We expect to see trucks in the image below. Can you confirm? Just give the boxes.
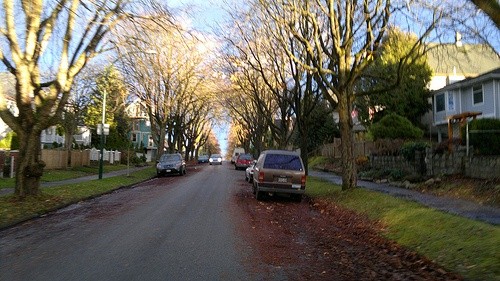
[231,148,245,164]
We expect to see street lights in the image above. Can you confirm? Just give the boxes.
[98,50,158,179]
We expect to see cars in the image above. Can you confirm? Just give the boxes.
[235,153,254,170]
[253,149,305,200]
[156,154,186,178]
[245,160,259,183]
[198,155,209,162]
[209,154,222,165]
